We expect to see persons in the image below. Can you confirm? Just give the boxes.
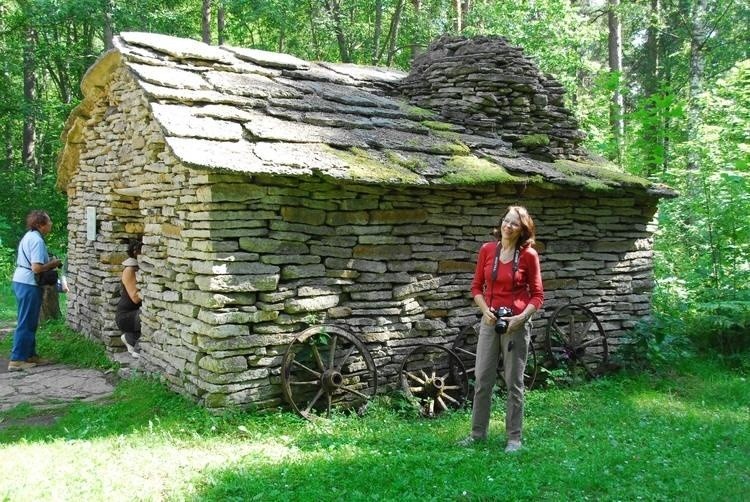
[7,209,61,371]
[114,237,144,359]
[454,204,545,454]
[61,252,69,293]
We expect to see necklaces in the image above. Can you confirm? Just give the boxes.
[499,246,513,261]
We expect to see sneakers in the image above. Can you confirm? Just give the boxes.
[7,356,49,372]
[121,333,141,359]
[503,440,523,455]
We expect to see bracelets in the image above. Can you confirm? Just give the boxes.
[522,310,530,320]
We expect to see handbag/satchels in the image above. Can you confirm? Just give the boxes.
[35,270,59,286]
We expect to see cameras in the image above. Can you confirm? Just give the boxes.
[494,306,514,334]
[56,256,64,269]
